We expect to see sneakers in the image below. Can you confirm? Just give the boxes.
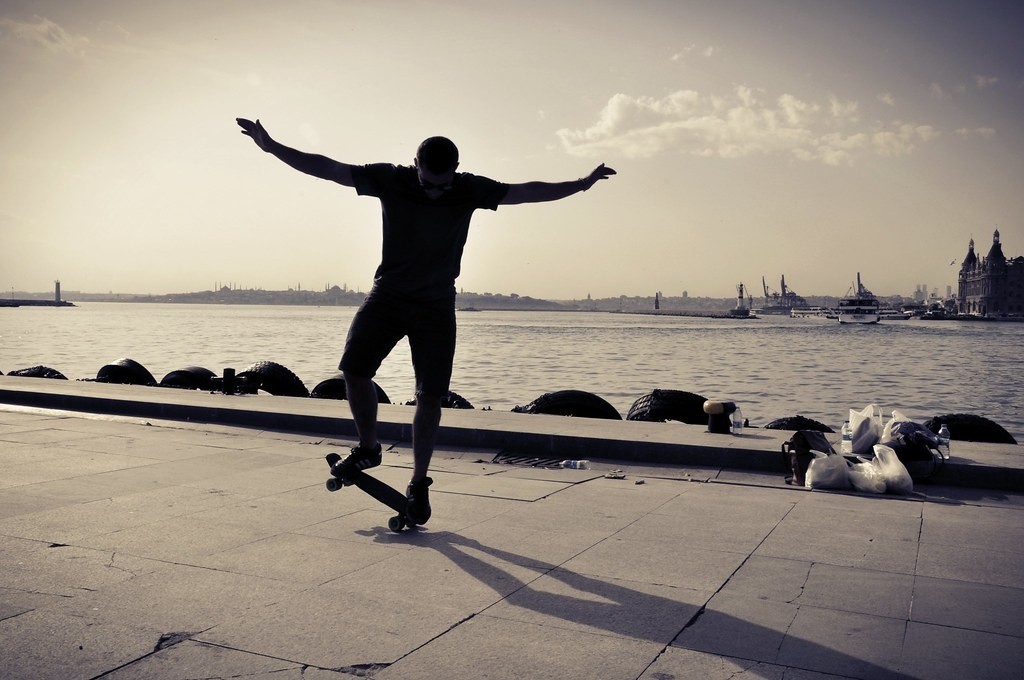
[406,477,433,523]
[330,443,382,478]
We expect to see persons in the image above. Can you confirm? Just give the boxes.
[237,118,620,522]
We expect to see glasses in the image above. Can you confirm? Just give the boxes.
[419,174,455,190]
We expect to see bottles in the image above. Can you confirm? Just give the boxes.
[558,460,591,471]
[732,407,743,435]
[937,424,950,459]
[841,421,853,453]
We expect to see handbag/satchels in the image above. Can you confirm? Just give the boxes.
[891,421,944,479]
[805,403,913,494]
[782,429,838,485]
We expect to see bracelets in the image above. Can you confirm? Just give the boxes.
[576,177,588,192]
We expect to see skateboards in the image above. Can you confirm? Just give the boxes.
[326,452,418,532]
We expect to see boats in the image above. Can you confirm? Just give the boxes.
[836,270,881,326]
[789,305,837,322]
[879,307,912,321]
